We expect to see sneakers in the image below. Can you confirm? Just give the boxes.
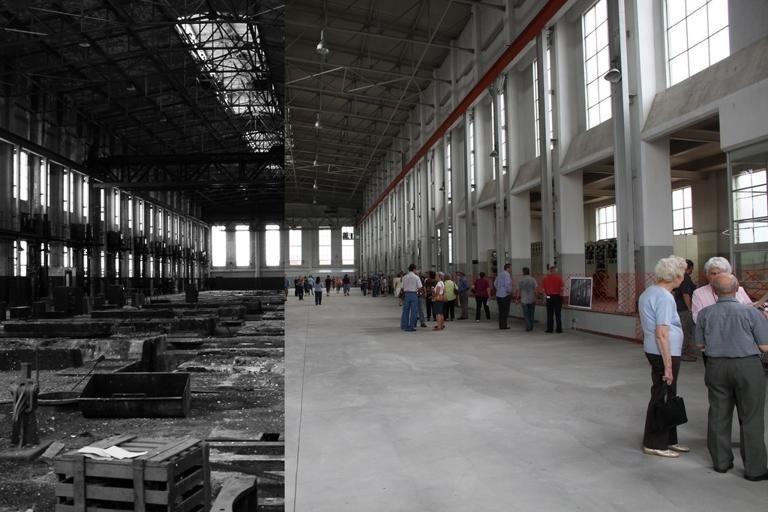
[412,318,444,331]
[500,327,511,329]
[446,317,466,321]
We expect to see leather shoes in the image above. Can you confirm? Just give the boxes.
[715,462,734,473]
[642,446,679,457]
[668,444,690,452]
[744,474,768,481]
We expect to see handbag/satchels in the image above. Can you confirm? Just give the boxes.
[432,294,448,302]
[652,396,688,431]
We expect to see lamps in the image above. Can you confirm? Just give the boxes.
[315,113,322,128]
[79,37,90,47]
[160,115,167,121]
[314,156,320,166]
[313,179,319,189]
[126,83,136,91]
[317,30,329,53]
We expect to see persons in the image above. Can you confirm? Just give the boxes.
[285,260,566,335]
[637,254,690,458]
[693,272,768,483]
[674,258,698,362]
[691,255,768,328]
